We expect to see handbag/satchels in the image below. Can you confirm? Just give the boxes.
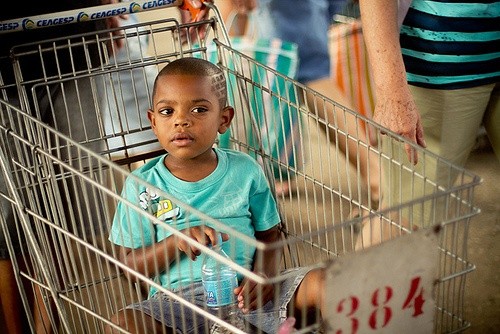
[197,7,306,180]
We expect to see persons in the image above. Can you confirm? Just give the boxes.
[230,0,386,233]
[0,0,210,334]
[354,0,500,251]
[105,56,328,334]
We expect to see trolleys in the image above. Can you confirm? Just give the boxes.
[0,0,486,334]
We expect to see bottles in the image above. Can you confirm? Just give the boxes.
[199,232,251,334]
